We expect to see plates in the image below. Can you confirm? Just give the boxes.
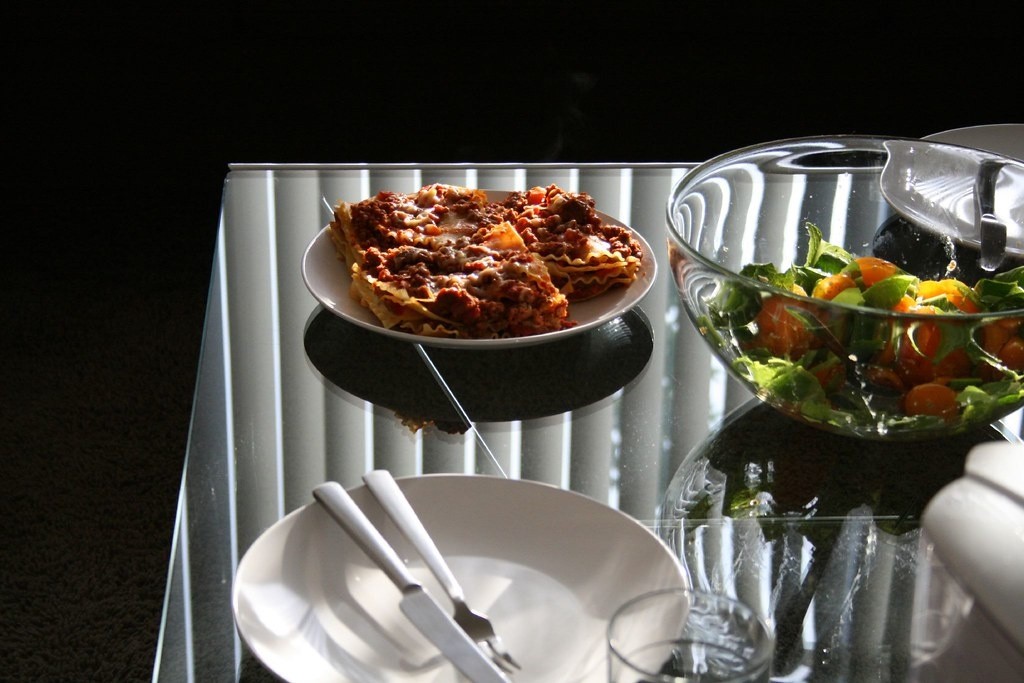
[230,474,687,682]
[303,191,657,345]
[880,122,1024,258]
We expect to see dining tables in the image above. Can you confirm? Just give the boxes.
[152,162,1023,683]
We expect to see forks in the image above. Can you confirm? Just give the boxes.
[362,470,520,675]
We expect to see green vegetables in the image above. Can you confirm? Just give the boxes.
[693,218,1024,443]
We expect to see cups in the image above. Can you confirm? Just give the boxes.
[607,588,772,682]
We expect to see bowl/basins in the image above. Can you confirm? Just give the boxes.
[666,136,1024,440]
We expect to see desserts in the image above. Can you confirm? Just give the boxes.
[325,184,647,340]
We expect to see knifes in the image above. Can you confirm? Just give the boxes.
[310,482,509,682]
[976,158,1007,272]
